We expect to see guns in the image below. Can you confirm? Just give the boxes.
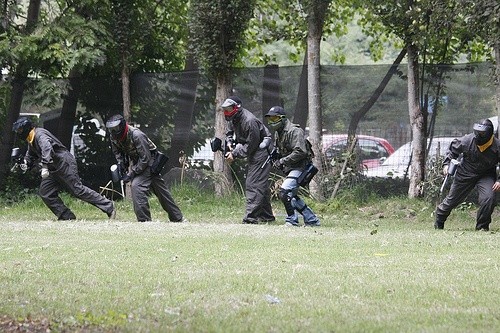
[110,157,125,198]
[439,158,459,193]
[258,135,277,169]
[211,130,236,159]
[9,147,32,175]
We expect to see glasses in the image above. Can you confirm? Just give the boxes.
[108,123,122,132]
[14,127,24,133]
[224,105,234,115]
[266,116,282,126]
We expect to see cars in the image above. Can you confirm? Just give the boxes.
[305,134,395,196]
[367,137,463,185]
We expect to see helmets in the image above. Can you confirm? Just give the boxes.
[11,117,34,141]
[222,97,242,121]
[266,106,286,131]
[106,114,126,139]
[473,119,493,146]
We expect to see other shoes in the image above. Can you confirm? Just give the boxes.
[304,221,321,227]
[109,201,116,219]
[434,220,444,230]
[285,222,293,226]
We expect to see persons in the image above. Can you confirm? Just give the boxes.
[11,117,116,221]
[211,96,275,224]
[259,106,320,227]
[106,114,188,223]
[433,118,500,230]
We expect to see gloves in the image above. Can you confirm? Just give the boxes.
[272,160,284,170]
[39,168,49,179]
[121,173,131,183]
